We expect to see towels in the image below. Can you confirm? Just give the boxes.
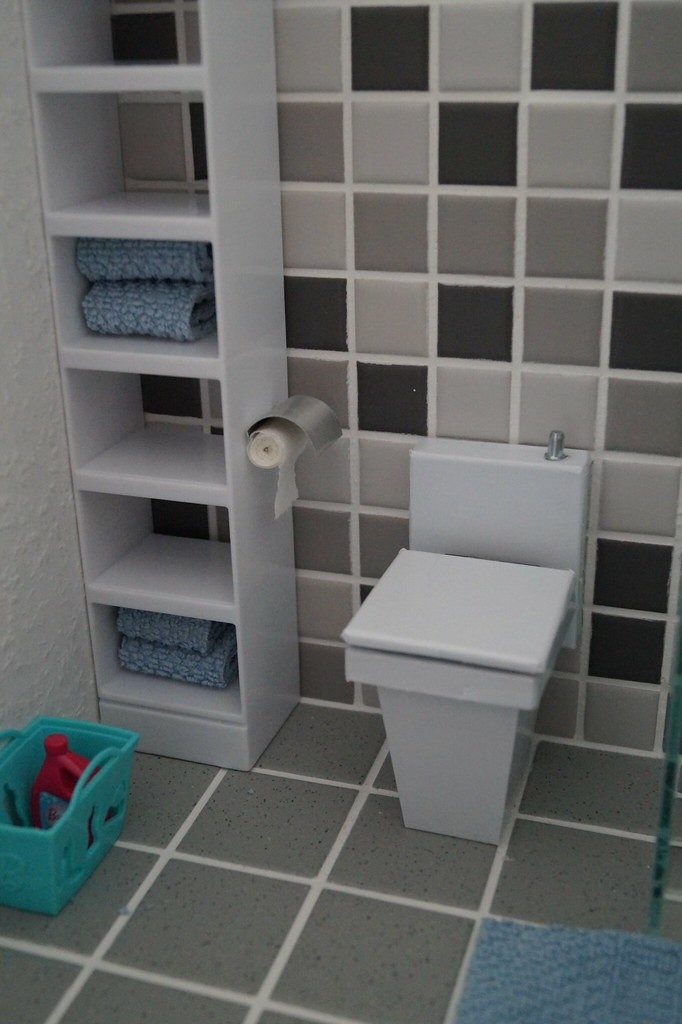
[80,279,217,344]
[115,607,228,655]
[116,625,239,690]
[75,237,215,284]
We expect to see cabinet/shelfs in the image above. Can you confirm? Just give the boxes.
[21,0,302,770]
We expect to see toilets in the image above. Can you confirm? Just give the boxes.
[339,437,592,847]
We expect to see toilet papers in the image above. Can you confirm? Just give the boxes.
[245,419,310,523]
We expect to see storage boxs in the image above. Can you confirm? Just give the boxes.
[0,716,139,915]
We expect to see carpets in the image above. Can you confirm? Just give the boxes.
[454,917,682,1024]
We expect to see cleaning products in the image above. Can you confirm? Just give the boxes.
[29,732,113,853]
